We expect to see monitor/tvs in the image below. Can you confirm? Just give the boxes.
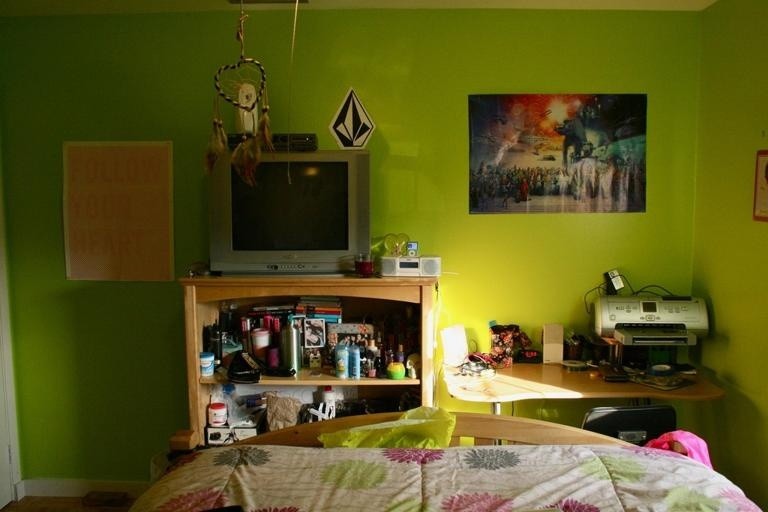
[208,150,371,278]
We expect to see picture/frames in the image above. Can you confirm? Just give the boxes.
[752,149,768,224]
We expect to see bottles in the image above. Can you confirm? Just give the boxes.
[335,330,405,381]
[322,385,335,419]
[207,403,227,426]
[250,313,302,372]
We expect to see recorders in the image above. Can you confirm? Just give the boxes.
[379,255,442,277]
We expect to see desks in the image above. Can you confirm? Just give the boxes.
[446,363,727,447]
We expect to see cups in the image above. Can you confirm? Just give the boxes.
[200,352,214,376]
[355,253,373,278]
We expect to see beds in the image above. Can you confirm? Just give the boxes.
[130,406,765,512]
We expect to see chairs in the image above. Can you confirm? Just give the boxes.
[581,404,679,448]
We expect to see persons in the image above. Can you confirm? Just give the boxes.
[469,101,646,213]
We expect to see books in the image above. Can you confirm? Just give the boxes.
[241,295,343,368]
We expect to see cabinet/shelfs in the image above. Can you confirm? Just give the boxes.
[177,273,439,447]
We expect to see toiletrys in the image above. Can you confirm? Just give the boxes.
[334,331,421,379]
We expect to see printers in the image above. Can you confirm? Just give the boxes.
[588,294,710,375]
